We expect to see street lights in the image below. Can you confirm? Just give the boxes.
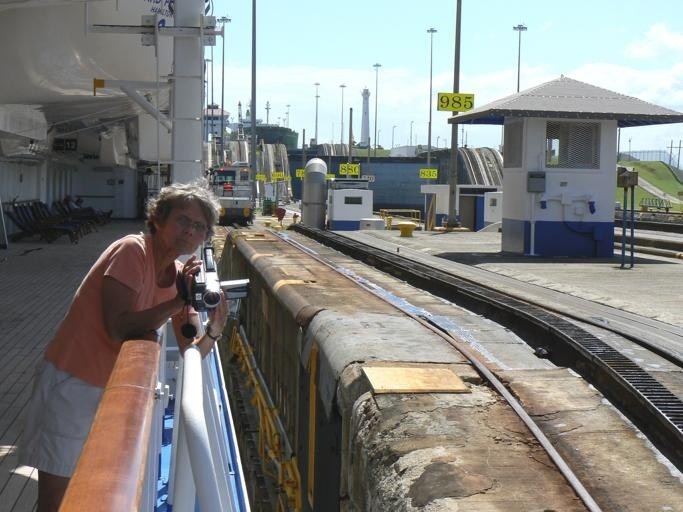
[512,24,527,93]
[410,120,414,146]
[217,15,233,166]
[372,63,382,156]
[286,105,291,128]
[339,84,347,155]
[205,58,212,143]
[424,26,438,230]
[377,129,381,145]
[392,125,398,148]
[314,82,321,144]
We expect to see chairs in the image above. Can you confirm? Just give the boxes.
[3,197,113,245]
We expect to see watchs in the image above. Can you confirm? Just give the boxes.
[206,329,226,343]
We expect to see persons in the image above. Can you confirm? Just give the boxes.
[16,180,231,512]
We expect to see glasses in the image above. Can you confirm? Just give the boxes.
[173,215,206,234]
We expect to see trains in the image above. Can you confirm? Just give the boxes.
[208,159,257,227]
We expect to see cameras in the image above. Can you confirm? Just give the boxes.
[192,246,251,311]
